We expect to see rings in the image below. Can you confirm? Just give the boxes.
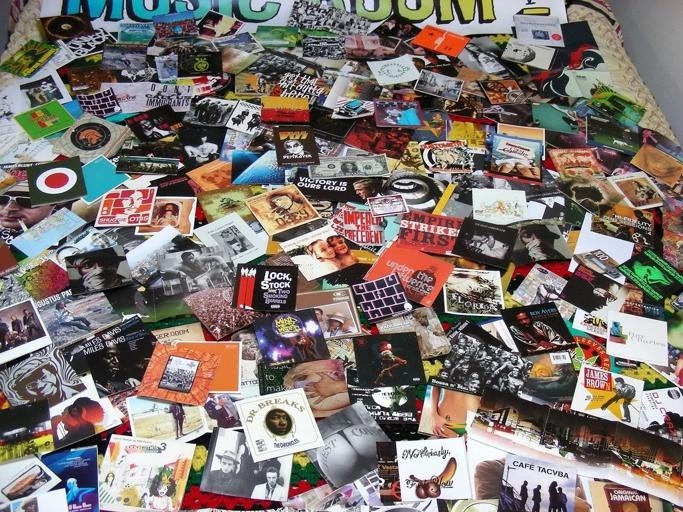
[439,427,444,431]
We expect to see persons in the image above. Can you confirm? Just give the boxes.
[434,83,447,96]
[204,392,239,428]
[335,162,363,176]
[231,114,245,124]
[327,236,359,267]
[242,110,249,117]
[98,473,118,504]
[510,311,566,350]
[77,249,119,290]
[602,378,636,422]
[29,86,48,103]
[431,387,480,438]
[251,112,259,118]
[631,180,658,203]
[22,310,41,339]
[88,339,141,395]
[51,407,95,448]
[232,331,260,383]
[406,265,436,303]
[157,203,178,228]
[268,192,312,222]
[184,130,218,165]
[10,357,76,411]
[257,73,267,93]
[243,83,254,93]
[325,313,348,337]
[64,480,94,507]
[40,81,56,99]
[519,481,529,510]
[248,118,260,130]
[411,310,446,356]
[398,24,412,36]
[220,230,246,256]
[265,409,288,437]
[549,482,559,510]
[10,316,29,337]
[134,286,147,315]
[285,139,311,162]
[433,337,533,398]
[381,19,396,34]
[3,328,19,340]
[513,223,567,263]
[0,95,13,120]
[315,309,329,335]
[135,114,169,138]
[307,240,338,272]
[207,450,244,497]
[147,475,176,511]
[56,246,77,266]
[55,301,97,334]
[555,488,566,511]
[386,104,402,124]
[426,54,449,66]
[251,467,283,501]
[166,252,232,289]
[357,338,405,382]
[165,402,185,438]
[0,191,57,230]
[648,411,683,442]
[464,43,511,80]
[483,81,518,101]
[194,99,234,124]
[113,191,147,226]
[412,57,425,74]
[353,180,381,204]
[532,485,542,512]
